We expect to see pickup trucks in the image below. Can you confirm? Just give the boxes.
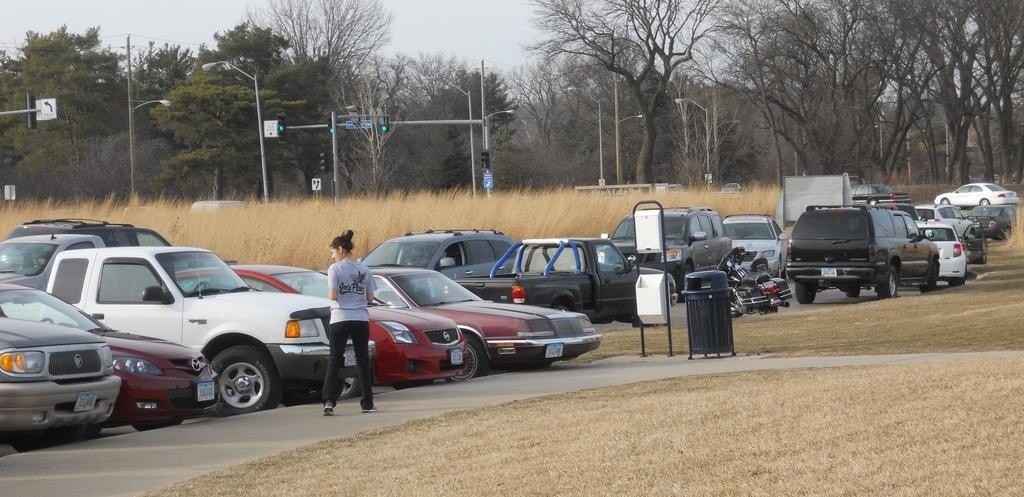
[454,236,679,327]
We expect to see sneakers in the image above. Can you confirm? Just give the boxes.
[361,404,378,413]
[324,400,334,416]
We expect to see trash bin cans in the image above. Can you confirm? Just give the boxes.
[681,269,737,360]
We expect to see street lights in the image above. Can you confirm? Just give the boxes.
[567,85,606,188]
[615,112,644,184]
[426,72,476,193]
[201,59,270,203]
[483,107,516,201]
[674,96,710,190]
[126,97,174,198]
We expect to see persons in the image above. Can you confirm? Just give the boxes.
[441,250,448,258]
[320,227,377,417]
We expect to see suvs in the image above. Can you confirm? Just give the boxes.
[597,204,733,302]
[789,193,989,304]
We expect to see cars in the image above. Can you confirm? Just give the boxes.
[722,212,789,280]
[964,204,1019,240]
[934,181,1019,208]
[851,184,895,202]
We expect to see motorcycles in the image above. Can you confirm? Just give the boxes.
[717,245,793,317]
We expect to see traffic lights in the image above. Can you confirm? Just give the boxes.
[327,116,333,134]
[378,115,389,133]
[320,149,327,173]
[480,149,490,170]
[279,124,286,134]
